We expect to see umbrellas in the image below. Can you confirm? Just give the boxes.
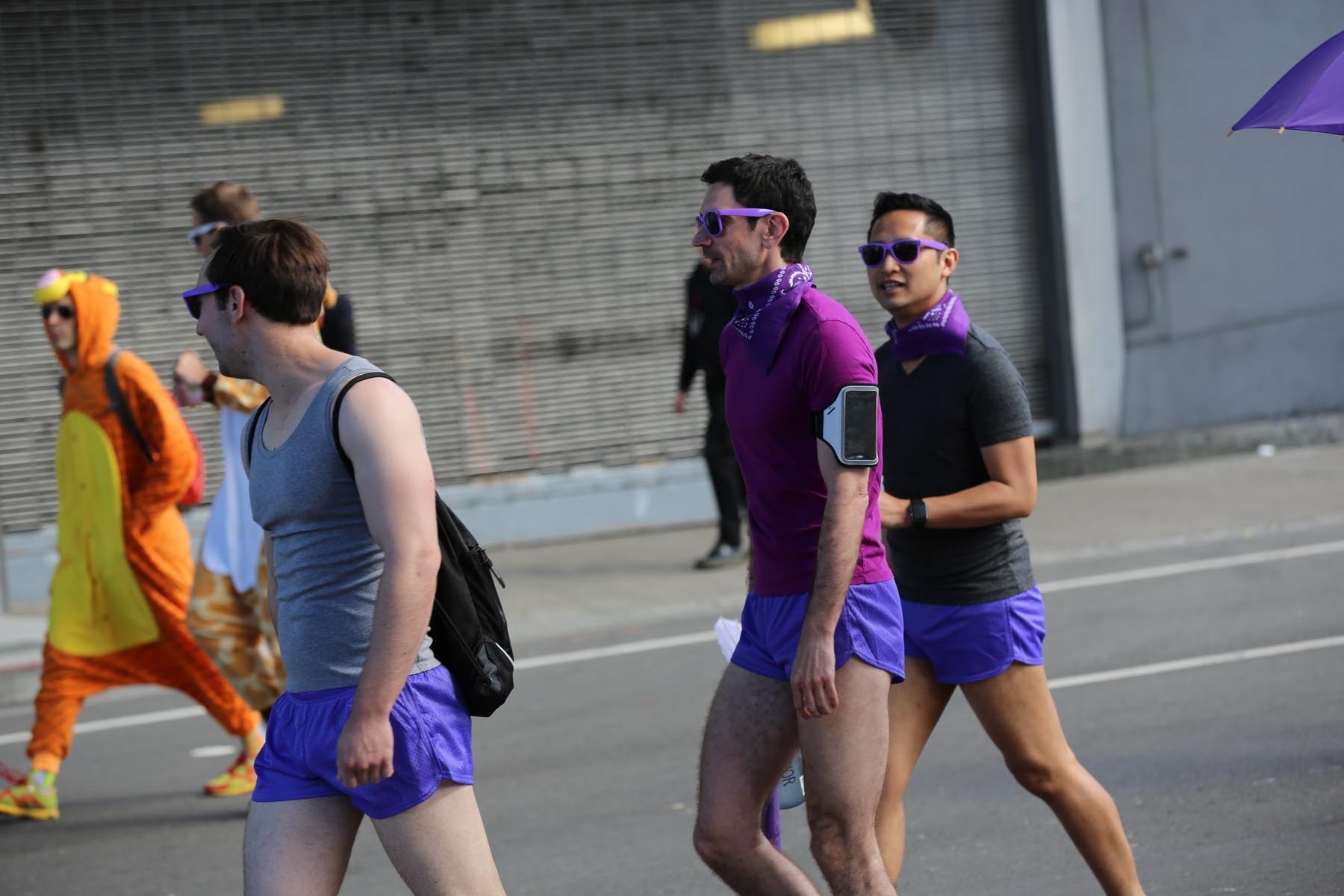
[1226,28,1344,140]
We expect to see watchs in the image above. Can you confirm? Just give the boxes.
[906,496,928,528]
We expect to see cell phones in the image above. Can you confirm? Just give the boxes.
[841,385,878,464]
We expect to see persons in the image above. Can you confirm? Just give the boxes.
[856,191,1146,896]
[181,217,514,896]
[0,268,268,821]
[674,242,749,569]
[174,181,341,717]
[685,153,904,896]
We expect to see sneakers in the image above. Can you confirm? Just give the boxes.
[1,763,59,821]
[206,753,259,797]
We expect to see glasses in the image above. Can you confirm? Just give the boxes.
[41,305,76,320]
[858,239,949,267]
[188,222,222,246]
[696,206,776,238]
[182,279,234,320]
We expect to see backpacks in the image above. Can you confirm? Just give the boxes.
[246,381,516,718]
[62,349,202,511]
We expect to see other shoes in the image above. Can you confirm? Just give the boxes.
[697,541,740,568]
